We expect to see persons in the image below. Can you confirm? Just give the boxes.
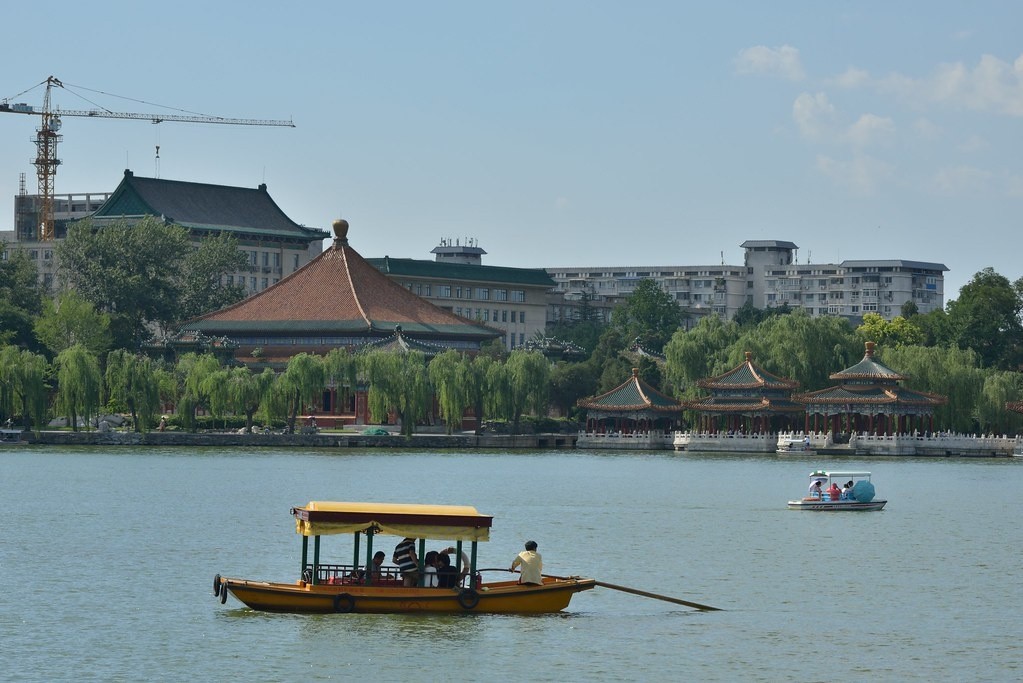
[825,482,840,502]
[805,435,812,451]
[844,480,856,500]
[429,551,440,567]
[726,422,747,440]
[506,539,547,586]
[418,551,438,592]
[158,416,167,434]
[436,554,463,589]
[840,484,849,498]
[236,414,323,439]
[920,428,951,441]
[814,480,823,500]
[390,534,426,585]
[7,416,16,430]
[362,551,394,582]
[804,436,807,441]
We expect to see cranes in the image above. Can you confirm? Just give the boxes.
[1,76,296,240]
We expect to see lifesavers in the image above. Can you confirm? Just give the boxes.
[802,497,820,501]
[440,547,470,579]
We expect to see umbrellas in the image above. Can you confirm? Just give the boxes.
[808,477,828,492]
[852,479,877,503]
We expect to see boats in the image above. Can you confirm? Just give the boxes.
[213,500,595,615]
[776,439,817,456]
[788,471,888,512]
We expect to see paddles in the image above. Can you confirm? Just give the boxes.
[476,568,722,611]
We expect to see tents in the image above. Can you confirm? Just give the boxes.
[292,498,496,588]
